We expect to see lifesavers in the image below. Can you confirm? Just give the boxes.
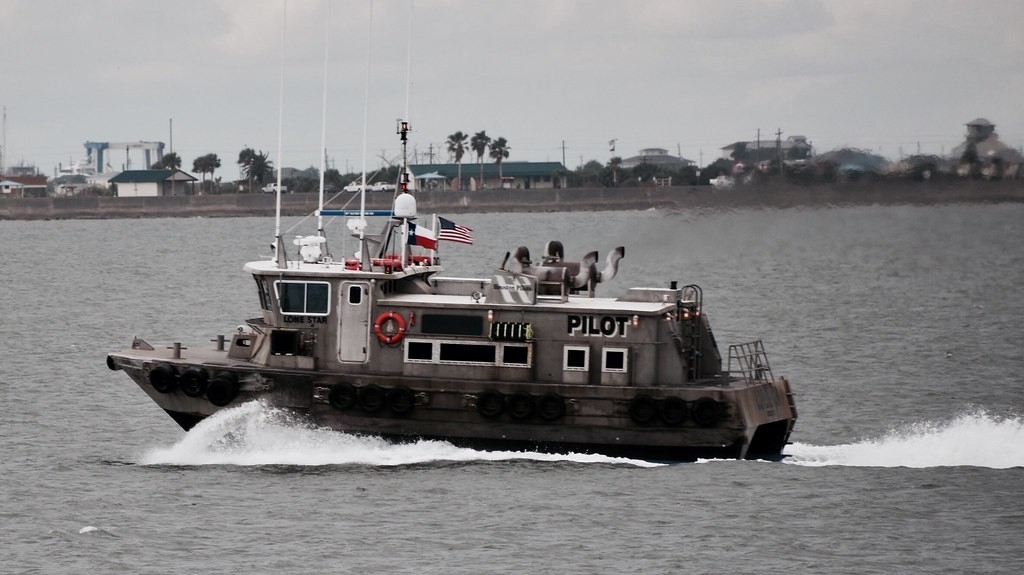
[345,260,359,270]
[372,258,401,267]
[374,311,406,344]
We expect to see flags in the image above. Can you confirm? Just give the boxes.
[407,221,437,250]
[438,216,473,244]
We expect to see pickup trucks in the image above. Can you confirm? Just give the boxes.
[344,182,372,192]
[372,182,395,192]
[261,183,288,194]
[709,174,734,185]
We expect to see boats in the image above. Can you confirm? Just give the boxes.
[105,119,798,464]
